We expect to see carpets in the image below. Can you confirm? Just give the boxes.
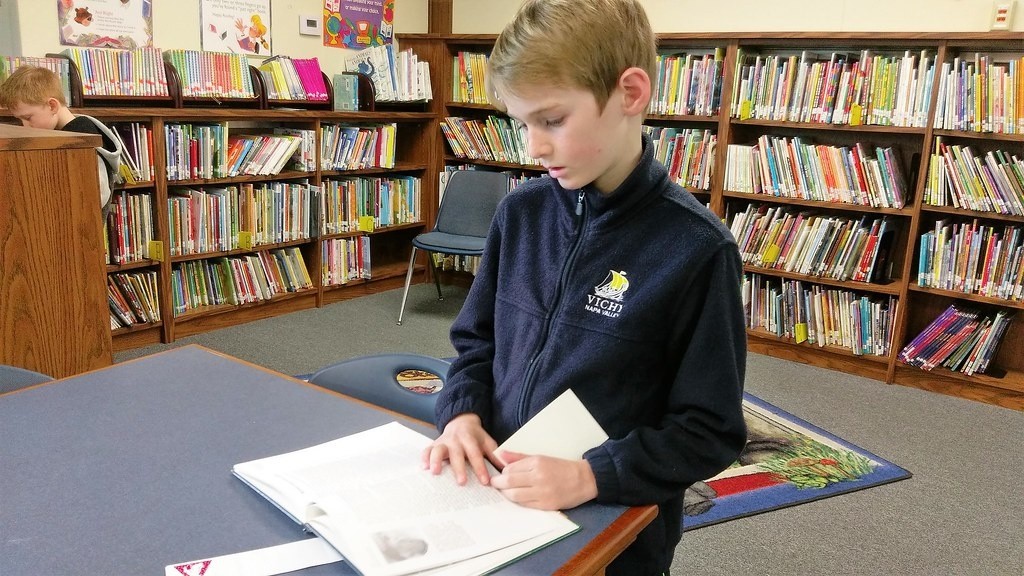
[292,355,912,533]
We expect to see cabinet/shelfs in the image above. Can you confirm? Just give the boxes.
[2,54,440,380]
[395,32,1024,410]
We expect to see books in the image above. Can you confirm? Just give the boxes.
[0,44,434,330]
[433,47,1024,377]
[229,420,583,576]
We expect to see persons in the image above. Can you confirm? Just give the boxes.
[422,0,747,576]
[0,66,122,227]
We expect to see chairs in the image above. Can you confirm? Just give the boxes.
[396,171,511,327]
[308,353,452,425]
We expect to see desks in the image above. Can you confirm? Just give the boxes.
[0,343,659,576]
